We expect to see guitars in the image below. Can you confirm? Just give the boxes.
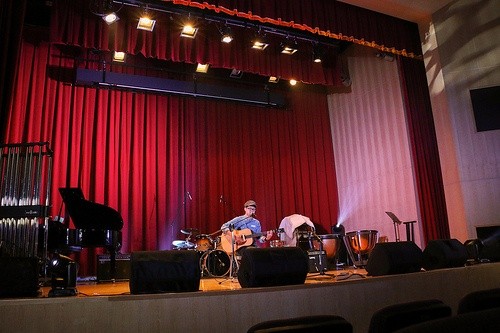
[221,228,284,253]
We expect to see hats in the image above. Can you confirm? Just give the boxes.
[243,200,256,207]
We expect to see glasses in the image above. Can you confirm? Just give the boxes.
[246,208,256,210]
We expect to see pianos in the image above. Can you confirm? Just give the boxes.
[38,187,124,284]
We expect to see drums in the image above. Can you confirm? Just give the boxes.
[346,230,378,255]
[217,236,236,254]
[270,240,286,248]
[200,249,231,278]
[310,234,341,260]
[195,234,212,251]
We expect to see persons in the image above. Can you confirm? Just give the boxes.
[221,200,276,255]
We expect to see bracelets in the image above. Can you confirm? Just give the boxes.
[265,237,267,241]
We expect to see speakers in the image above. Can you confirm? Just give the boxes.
[96,255,131,281]
[236,247,308,288]
[365,241,423,277]
[128,249,201,296]
[421,238,468,270]
[0,255,41,298]
[469,85,500,132]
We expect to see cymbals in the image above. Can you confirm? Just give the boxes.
[181,229,200,234]
[172,240,194,248]
